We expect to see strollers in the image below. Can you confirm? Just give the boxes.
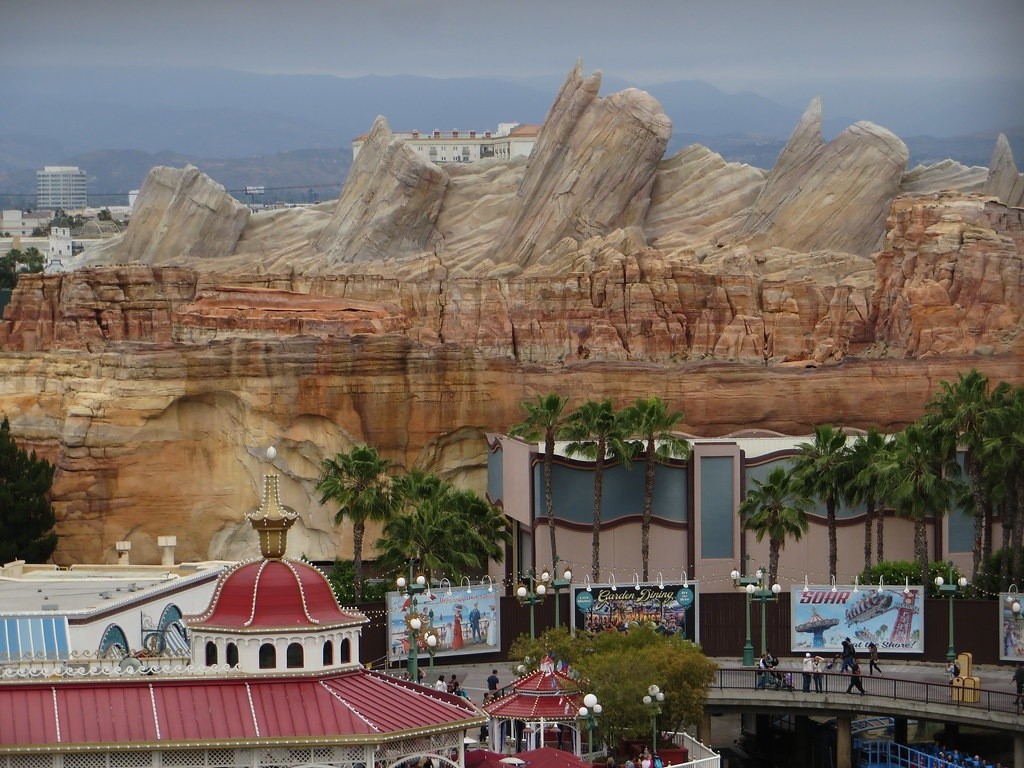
[458,688,478,707]
[769,667,795,692]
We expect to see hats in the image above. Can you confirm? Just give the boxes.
[806,653,810,656]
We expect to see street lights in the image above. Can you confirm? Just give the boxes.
[751,561,783,658]
[643,684,666,753]
[935,566,969,673]
[397,556,422,685]
[578,693,604,764]
[543,554,574,629]
[731,552,765,666]
[517,566,546,642]
[424,630,440,688]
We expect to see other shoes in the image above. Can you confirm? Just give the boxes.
[880,673,883,677]
[1013,701,1018,704]
[868,674,872,676]
[846,691,851,694]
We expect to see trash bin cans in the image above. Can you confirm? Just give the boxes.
[952,676,966,702]
[963,677,981,704]
[958,652,972,678]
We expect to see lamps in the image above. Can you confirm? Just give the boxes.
[583,575,591,592]
[803,575,910,593]
[405,575,494,599]
[633,573,640,590]
[609,573,617,591]
[656,571,665,589]
[681,571,688,588]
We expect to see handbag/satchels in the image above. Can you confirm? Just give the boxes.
[874,659,880,664]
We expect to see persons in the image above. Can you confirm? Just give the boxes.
[946,660,954,697]
[404,671,411,681]
[802,653,825,693]
[912,740,1001,768]
[605,757,616,768]
[436,674,460,696]
[1009,663,1024,704]
[624,753,662,768]
[421,603,498,649]
[483,692,489,705]
[866,643,883,677]
[487,669,499,689]
[418,668,426,683]
[846,660,864,696]
[839,638,856,673]
[757,649,773,691]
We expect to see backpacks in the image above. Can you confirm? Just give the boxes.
[949,664,960,677]
[770,654,779,666]
[757,660,763,675]
